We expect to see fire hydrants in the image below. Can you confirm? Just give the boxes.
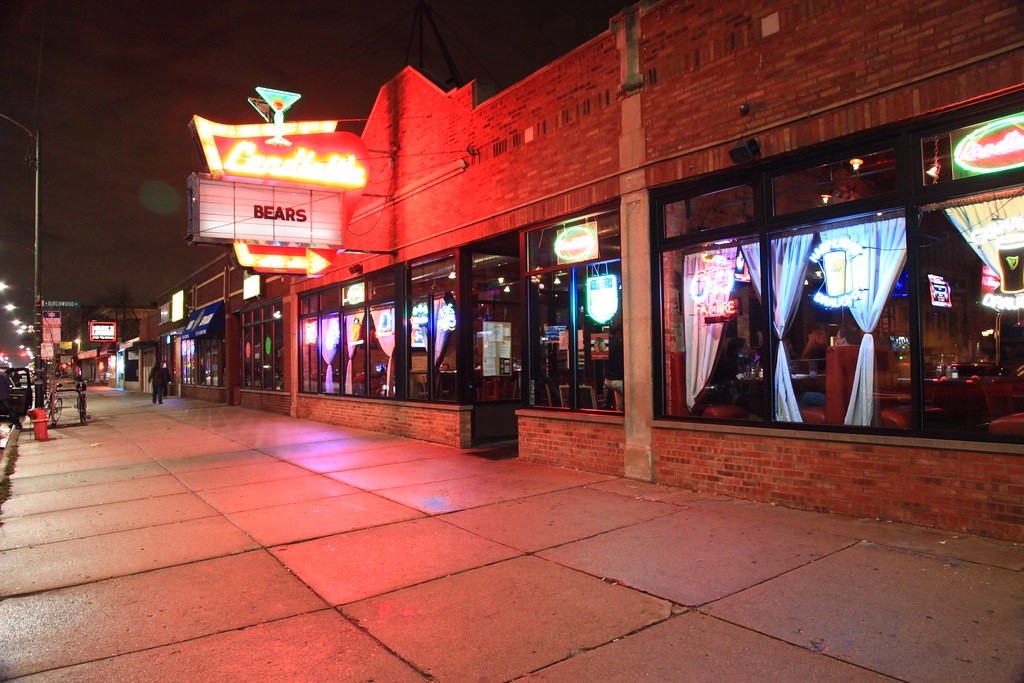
[28,405,52,440]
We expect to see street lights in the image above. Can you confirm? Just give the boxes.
[0,279,44,367]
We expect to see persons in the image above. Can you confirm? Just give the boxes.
[437,318,858,398]
[160,360,171,397]
[147,362,165,404]
[5,367,23,429]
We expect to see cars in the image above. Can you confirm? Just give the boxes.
[0,367,34,423]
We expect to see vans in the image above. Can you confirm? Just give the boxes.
[946,358,1006,381]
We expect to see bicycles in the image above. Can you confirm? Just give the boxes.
[41,379,64,425]
[68,379,95,424]
